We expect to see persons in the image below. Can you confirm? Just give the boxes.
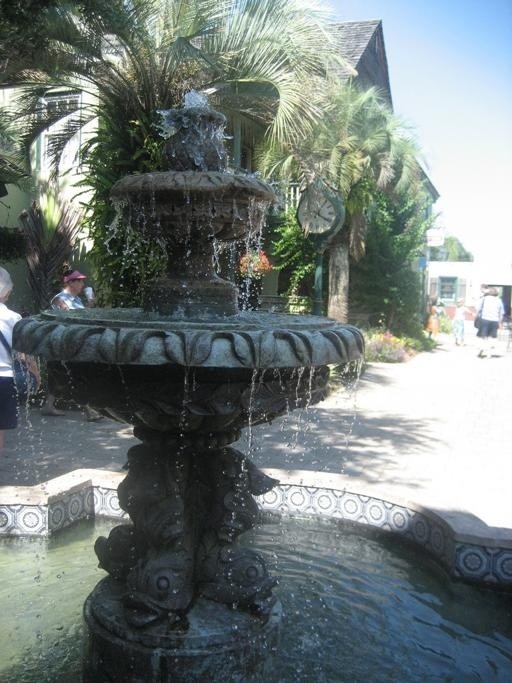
[425,284,505,358]
[50,269,101,421]
[0,267,42,430]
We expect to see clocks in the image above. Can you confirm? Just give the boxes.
[295,172,346,255]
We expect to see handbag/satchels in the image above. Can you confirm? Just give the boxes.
[473,314,482,328]
[11,358,37,395]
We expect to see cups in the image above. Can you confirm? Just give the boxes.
[83,284,95,303]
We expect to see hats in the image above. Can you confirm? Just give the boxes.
[62,271,86,283]
